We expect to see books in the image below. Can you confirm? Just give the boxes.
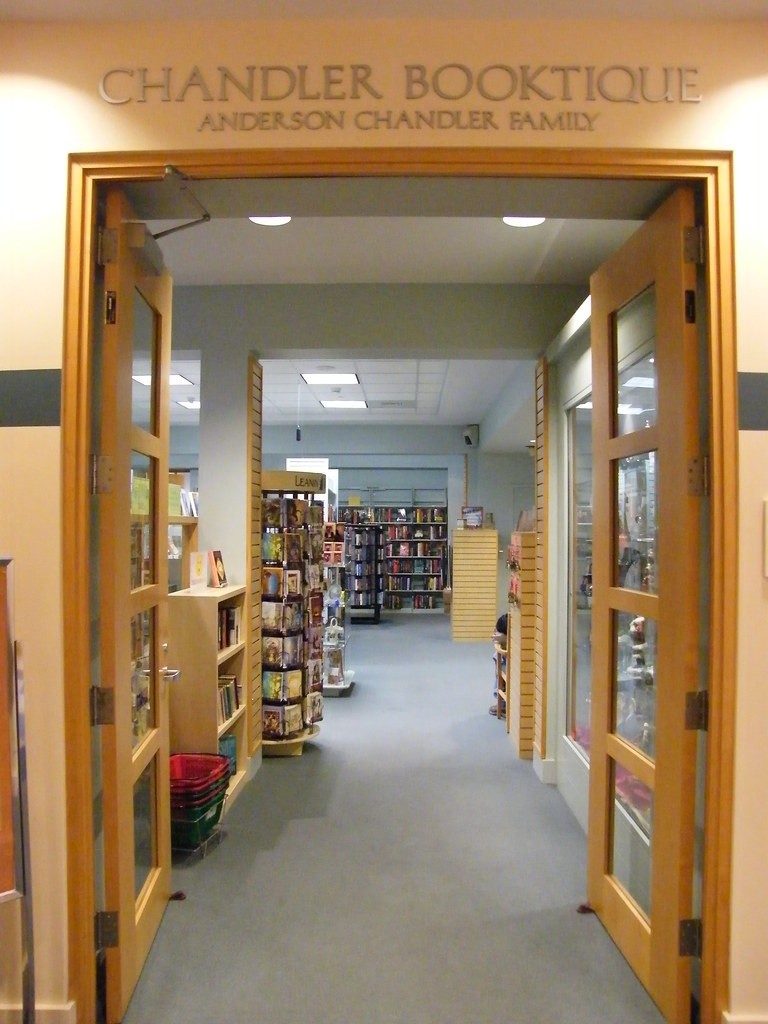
[261,500,323,736]
[324,506,448,610]
[219,607,239,775]
[130,524,154,749]
[129,475,199,518]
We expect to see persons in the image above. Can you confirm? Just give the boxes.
[489,613,508,715]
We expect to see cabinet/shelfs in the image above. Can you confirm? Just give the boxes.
[452,523,546,758]
[127,464,453,829]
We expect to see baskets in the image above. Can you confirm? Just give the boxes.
[169,752,231,851]
